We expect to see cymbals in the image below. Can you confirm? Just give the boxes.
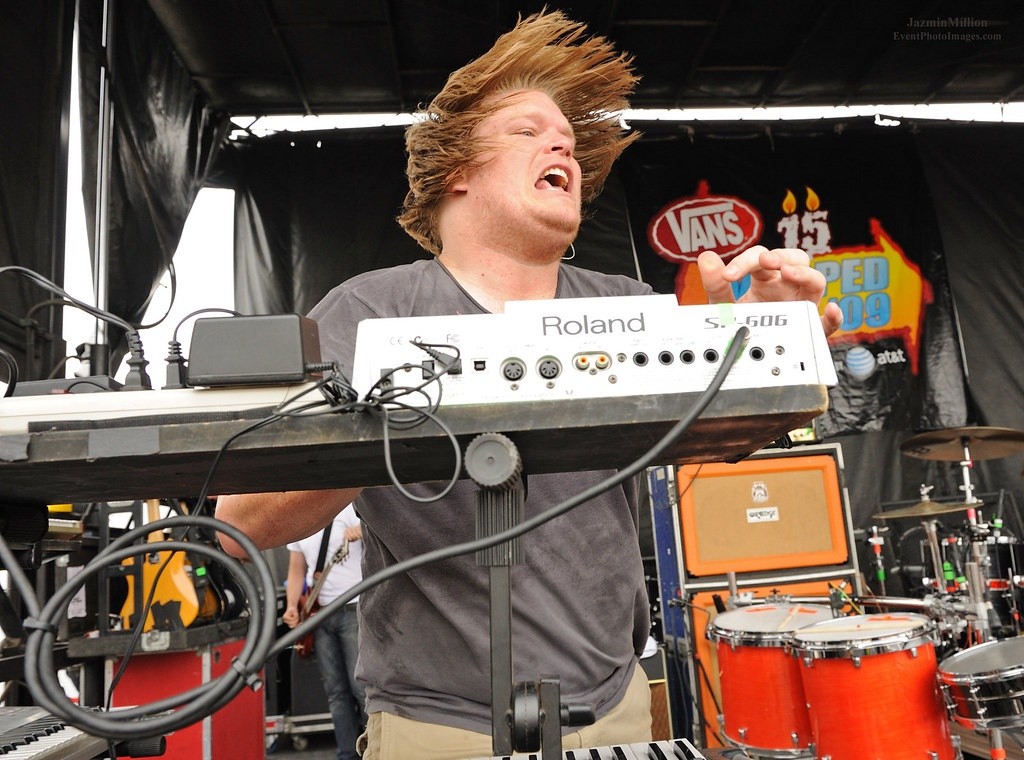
[899,426,1024,461]
[873,501,985,519]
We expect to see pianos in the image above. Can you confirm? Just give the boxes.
[463,736,752,760]
[1,702,141,760]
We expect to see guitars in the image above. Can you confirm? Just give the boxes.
[119,499,249,633]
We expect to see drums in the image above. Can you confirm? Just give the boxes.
[791,612,957,760]
[934,634,1024,731]
[707,603,848,757]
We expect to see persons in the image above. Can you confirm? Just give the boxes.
[213,15,842,760]
[282,501,371,760]
